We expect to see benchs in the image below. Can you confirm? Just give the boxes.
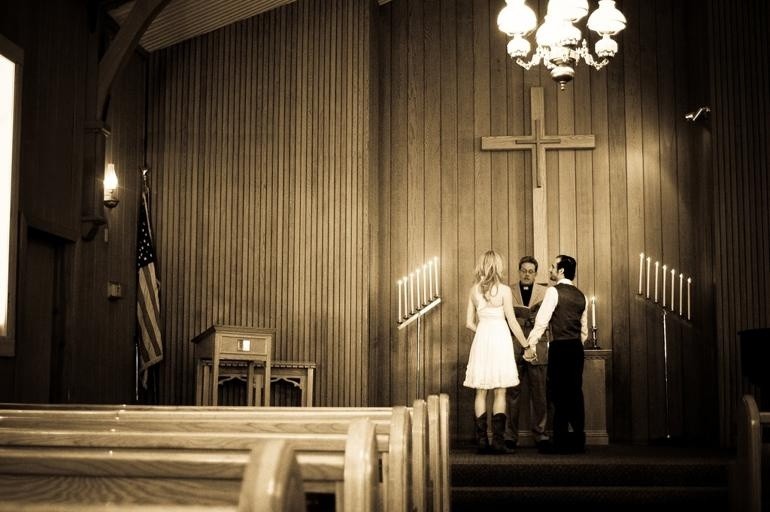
[0,359,451,512]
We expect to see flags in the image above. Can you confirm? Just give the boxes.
[136,185,164,390]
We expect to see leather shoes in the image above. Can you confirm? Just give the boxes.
[539,440,585,454]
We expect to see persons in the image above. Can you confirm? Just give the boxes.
[463,250,535,454]
[523,255,589,455]
[503,256,550,448]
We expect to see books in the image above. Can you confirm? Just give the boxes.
[513,300,542,319]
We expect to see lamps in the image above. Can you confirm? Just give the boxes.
[497,0,628,91]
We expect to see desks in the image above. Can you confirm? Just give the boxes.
[190,325,275,406]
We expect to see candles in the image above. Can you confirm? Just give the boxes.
[589,252,694,330]
[395,254,440,322]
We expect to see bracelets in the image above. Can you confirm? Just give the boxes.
[523,345,530,350]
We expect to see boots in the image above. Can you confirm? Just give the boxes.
[473,411,491,454]
[491,412,516,455]
